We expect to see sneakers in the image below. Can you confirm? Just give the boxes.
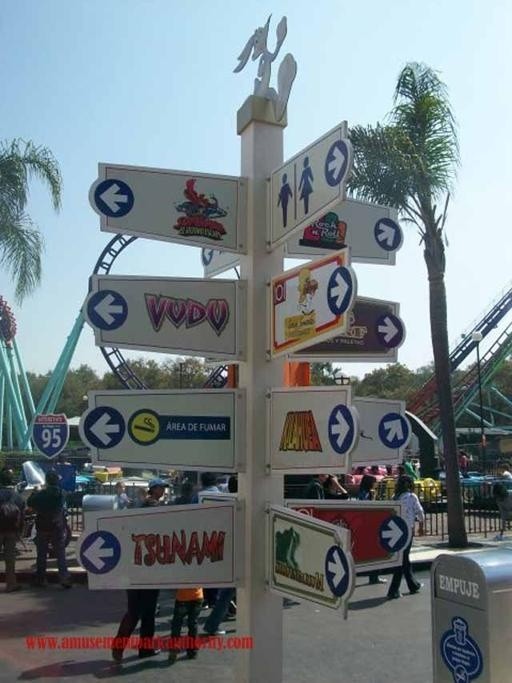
[369,577,425,600]
[110,600,237,665]
[5,577,73,593]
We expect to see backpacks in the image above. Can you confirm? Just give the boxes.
[0,490,22,533]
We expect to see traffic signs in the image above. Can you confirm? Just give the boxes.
[31,413,70,460]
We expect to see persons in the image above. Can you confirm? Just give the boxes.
[165,588,205,664]
[493,482,512,540]
[202,588,237,638]
[356,474,388,584]
[202,588,218,610]
[138,479,170,506]
[104,588,163,664]
[502,462,511,480]
[167,481,194,506]
[385,476,425,598]
[322,475,349,499]
[113,481,129,508]
[370,465,379,475]
[189,471,221,504]
[0,466,28,593]
[396,465,406,478]
[28,471,74,590]
[226,476,238,492]
[458,449,468,472]
[301,474,327,499]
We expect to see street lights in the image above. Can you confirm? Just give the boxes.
[473,329,489,477]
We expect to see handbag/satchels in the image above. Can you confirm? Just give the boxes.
[35,520,59,538]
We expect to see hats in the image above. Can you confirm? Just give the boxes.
[148,477,170,488]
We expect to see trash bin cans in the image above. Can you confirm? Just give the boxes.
[82,494,117,532]
[430,549,512,683]
